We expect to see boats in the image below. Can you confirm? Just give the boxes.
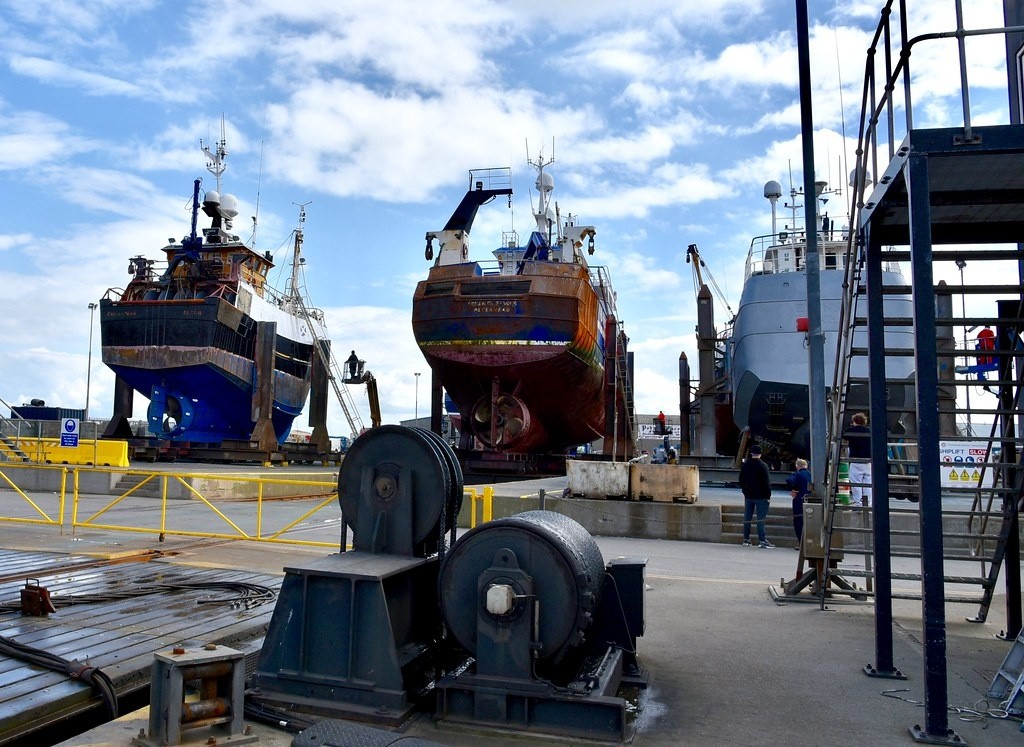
[413,133,629,459]
[719,153,915,463]
[99,109,326,445]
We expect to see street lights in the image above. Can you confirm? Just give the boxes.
[954,260,973,438]
[85,303,100,422]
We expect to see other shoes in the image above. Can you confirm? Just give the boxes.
[794,546,800,551]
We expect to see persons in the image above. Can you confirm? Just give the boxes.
[653,446,676,465]
[738,446,777,549]
[656,411,665,435]
[844,414,873,507]
[977,325,995,365]
[346,351,359,379]
[786,458,812,551]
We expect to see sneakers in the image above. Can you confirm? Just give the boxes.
[759,539,776,548]
[743,539,752,547]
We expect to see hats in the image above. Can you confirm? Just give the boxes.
[750,445,762,455]
